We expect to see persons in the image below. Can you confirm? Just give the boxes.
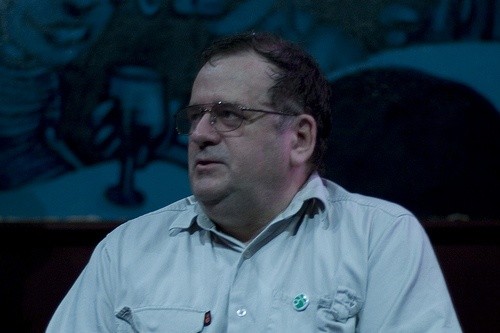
[45,31,464,333]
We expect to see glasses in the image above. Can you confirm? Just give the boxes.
[175,103,299,136]
